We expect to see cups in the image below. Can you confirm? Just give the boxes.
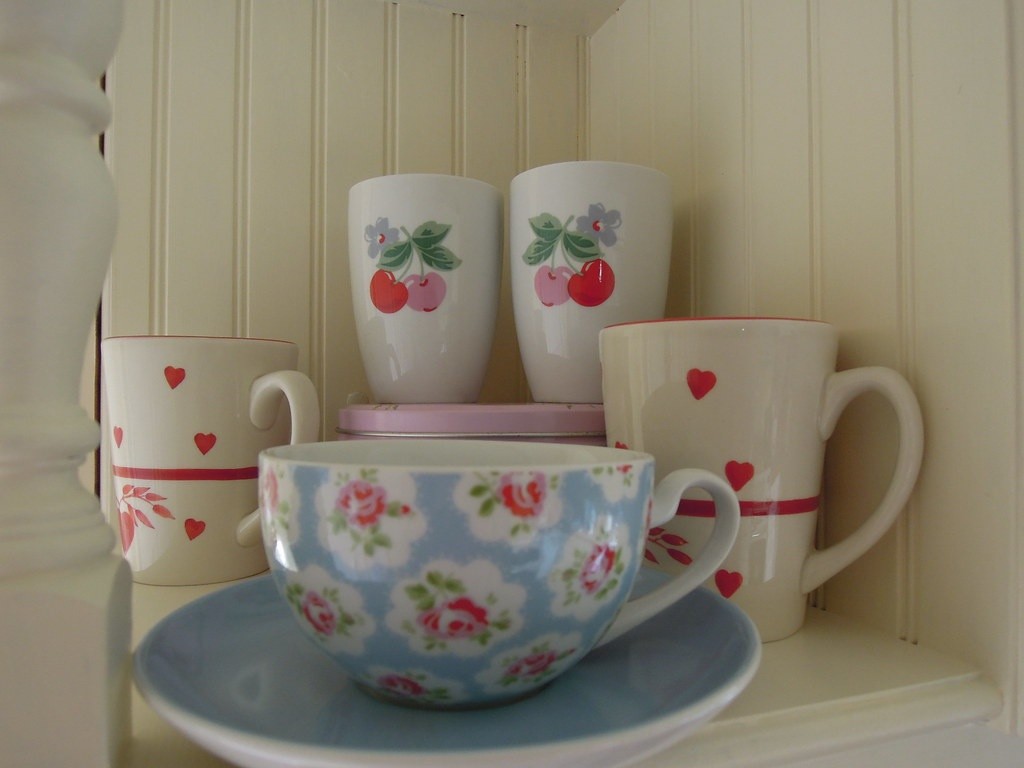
[99,336,323,586]
[254,437,743,712]
[599,317,923,645]
[507,159,673,403]
[346,173,503,405]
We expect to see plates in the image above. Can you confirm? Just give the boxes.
[132,565,763,768]
